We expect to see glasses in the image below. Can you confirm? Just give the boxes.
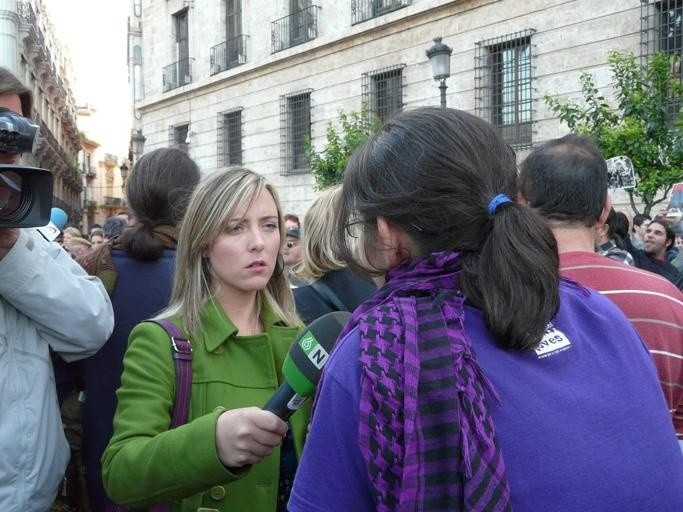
[345,218,423,238]
[284,241,294,247]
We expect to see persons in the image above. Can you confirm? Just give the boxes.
[284,105,683,512]
[1,66,115,510]
[46,134,682,511]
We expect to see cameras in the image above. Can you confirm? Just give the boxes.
[0,107,53,228]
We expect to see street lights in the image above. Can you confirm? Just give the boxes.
[424,36,454,108]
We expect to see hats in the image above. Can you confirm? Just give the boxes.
[285,227,300,238]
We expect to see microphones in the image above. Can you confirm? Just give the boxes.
[261,311,352,422]
